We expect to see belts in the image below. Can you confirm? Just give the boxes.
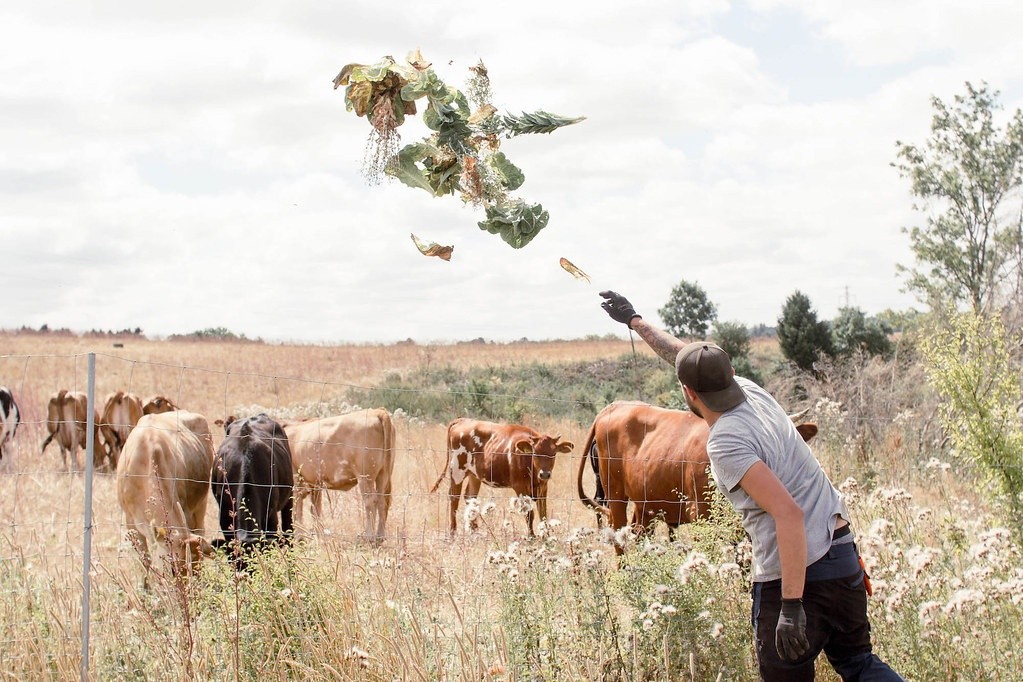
[832,522,851,539]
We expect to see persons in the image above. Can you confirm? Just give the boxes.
[597,288,900,682]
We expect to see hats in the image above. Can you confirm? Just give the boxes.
[675,342,747,413]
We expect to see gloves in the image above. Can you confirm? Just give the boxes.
[599,290,642,329]
[776,599,810,660]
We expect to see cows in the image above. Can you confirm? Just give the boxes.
[39,390,395,587]
[0,385,20,461]
[429,418,575,536]
[577,400,818,571]
